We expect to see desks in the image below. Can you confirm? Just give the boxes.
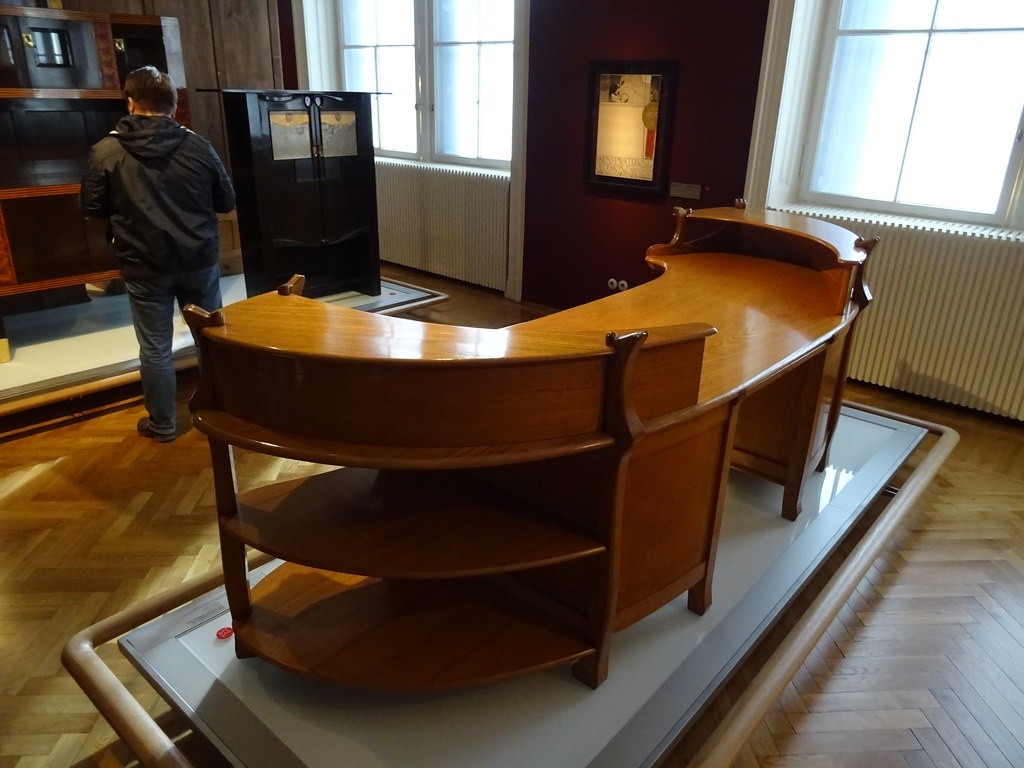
[183,199,882,693]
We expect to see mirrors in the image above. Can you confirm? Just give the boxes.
[581,58,681,196]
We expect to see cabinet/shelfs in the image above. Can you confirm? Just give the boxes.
[195,88,393,300]
[0,5,193,316]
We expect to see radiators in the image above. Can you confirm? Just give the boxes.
[373,156,511,292]
[766,207,1024,424]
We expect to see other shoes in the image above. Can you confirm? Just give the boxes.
[137,417,176,441]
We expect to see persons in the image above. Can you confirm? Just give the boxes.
[79,66,235,442]
